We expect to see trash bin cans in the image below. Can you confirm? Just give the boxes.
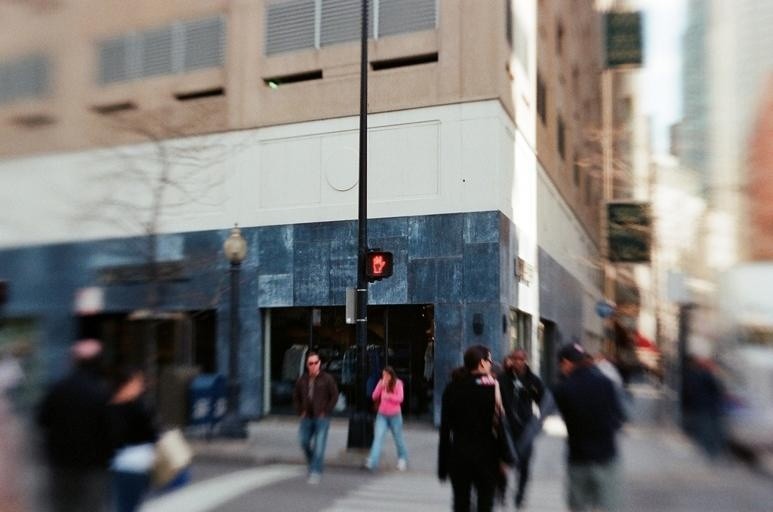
[189,373,228,431]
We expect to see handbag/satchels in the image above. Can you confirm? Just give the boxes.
[148,430,192,487]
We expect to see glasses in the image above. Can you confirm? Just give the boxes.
[485,357,494,367]
[309,359,320,365]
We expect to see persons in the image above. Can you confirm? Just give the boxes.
[438,341,629,511]
[33,335,147,510]
[678,353,724,461]
[105,355,158,510]
[363,365,409,473]
[292,349,340,486]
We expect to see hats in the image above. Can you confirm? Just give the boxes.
[558,343,591,361]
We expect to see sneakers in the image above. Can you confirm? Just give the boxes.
[307,473,322,485]
[395,458,408,469]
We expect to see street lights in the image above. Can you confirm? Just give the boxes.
[218,217,250,441]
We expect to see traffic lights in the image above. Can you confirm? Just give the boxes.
[363,249,395,280]
